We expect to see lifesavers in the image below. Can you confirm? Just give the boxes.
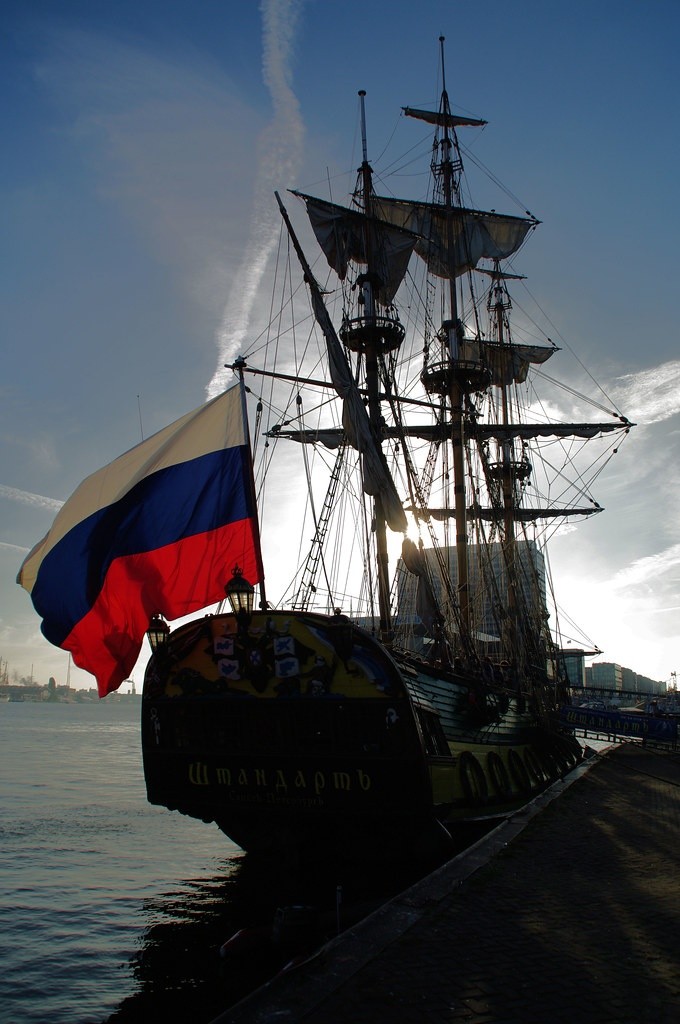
[460,750,489,811]
[507,748,532,796]
[486,752,513,802]
[524,747,545,785]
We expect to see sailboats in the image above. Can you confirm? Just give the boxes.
[138,31,633,879]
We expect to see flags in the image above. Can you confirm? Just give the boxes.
[17,380,266,700]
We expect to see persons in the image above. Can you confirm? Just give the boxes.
[395,648,442,669]
